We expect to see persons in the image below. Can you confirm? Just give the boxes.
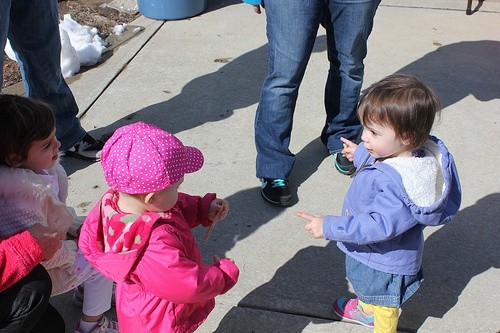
[0,94,120,333]
[244,0,382,206]
[0,1,106,161]
[78,121,240,333]
[297,75,461,333]
[0,224,65,333]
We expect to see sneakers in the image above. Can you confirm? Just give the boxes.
[62,133,105,161]
[332,297,374,328]
[261,177,292,204]
[75,314,118,333]
[73,283,116,306]
[332,151,357,174]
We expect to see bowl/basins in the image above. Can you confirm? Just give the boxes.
[137,0,206,20]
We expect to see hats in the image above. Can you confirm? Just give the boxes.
[100,121,204,194]
